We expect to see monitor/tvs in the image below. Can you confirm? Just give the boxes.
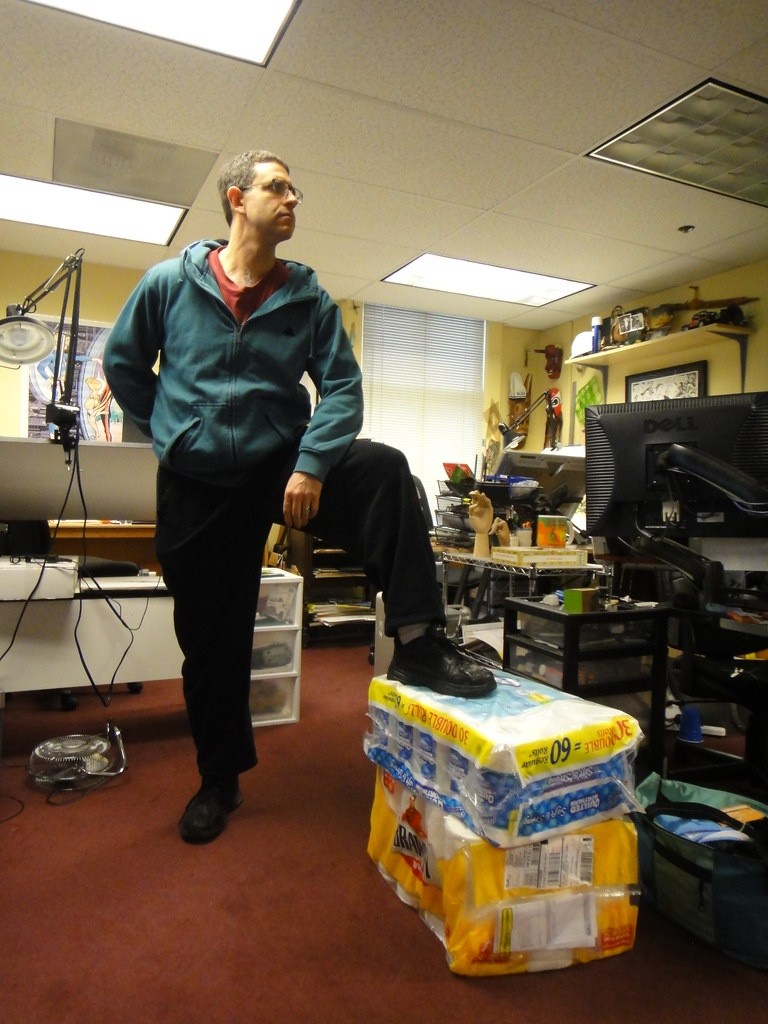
[585,392,768,538]
[0,519,50,557]
[495,450,586,529]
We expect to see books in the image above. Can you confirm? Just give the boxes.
[308,600,376,626]
[313,568,366,578]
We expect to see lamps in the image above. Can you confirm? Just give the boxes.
[663,700,704,744]
[0,244,91,466]
[496,391,564,453]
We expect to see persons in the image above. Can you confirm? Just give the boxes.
[100,151,497,844]
[489,517,510,546]
[468,490,494,557]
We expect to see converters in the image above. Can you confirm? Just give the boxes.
[25,554,58,564]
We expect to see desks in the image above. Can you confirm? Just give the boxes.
[47,517,158,539]
[0,566,188,731]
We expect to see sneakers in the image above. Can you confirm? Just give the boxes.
[178,777,243,845]
[387,628,496,696]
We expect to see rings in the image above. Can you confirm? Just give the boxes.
[306,506,310,511]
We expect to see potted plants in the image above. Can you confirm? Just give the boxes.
[516,521,534,548]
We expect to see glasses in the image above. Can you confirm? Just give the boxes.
[241,178,303,205]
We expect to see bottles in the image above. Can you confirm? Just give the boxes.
[591,317,603,354]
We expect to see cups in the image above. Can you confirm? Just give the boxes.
[516,527,533,546]
[536,515,574,548]
[678,704,704,743]
[510,536,519,547]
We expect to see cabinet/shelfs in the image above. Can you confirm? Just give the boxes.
[249,566,305,730]
[505,594,671,782]
[289,527,381,649]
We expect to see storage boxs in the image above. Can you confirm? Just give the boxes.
[490,544,589,569]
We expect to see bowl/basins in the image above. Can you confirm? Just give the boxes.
[589,536,611,565]
[543,595,559,605]
[444,474,544,503]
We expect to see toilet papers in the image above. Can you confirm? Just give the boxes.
[362,667,647,978]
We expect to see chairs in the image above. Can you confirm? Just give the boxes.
[412,473,492,621]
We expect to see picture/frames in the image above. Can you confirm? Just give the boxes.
[626,357,707,405]
